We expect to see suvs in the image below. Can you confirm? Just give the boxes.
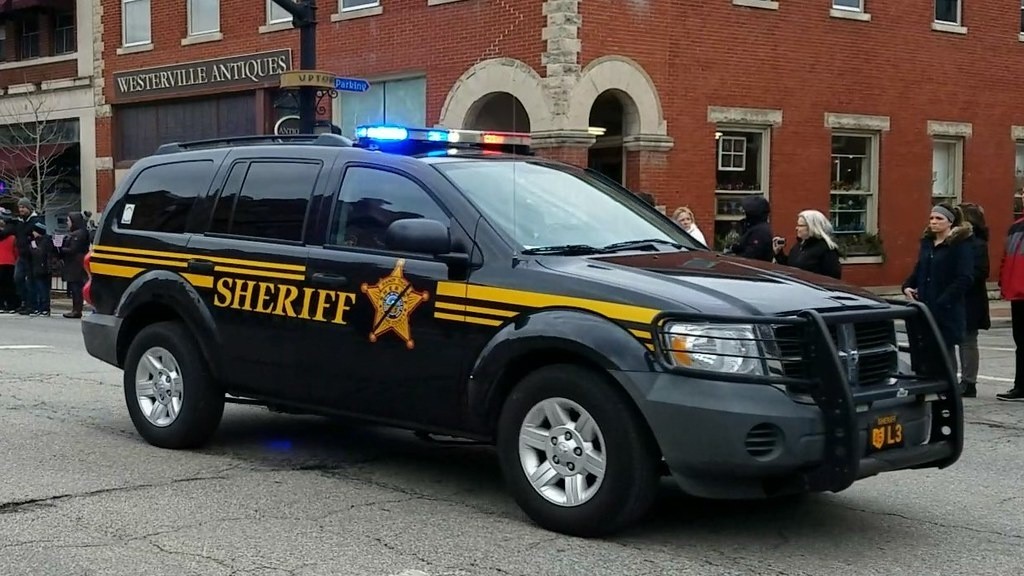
[82,122,968,538]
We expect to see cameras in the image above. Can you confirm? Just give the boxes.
[777,239,786,246]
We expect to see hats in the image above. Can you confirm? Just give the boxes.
[17,197,35,210]
[81,210,92,226]
[32,222,47,235]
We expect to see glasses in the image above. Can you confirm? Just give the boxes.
[797,224,808,229]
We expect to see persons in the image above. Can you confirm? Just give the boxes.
[996,191,1024,401]
[61,212,90,318]
[673,207,707,248]
[637,192,655,207]
[725,196,773,263]
[773,209,841,281]
[0,199,51,317]
[957,204,991,397]
[901,203,973,378]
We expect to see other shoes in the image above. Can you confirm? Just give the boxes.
[40,310,50,318]
[0,308,33,315]
[63,311,82,319]
[996,389,1024,401]
[957,380,976,398]
[30,310,42,316]
